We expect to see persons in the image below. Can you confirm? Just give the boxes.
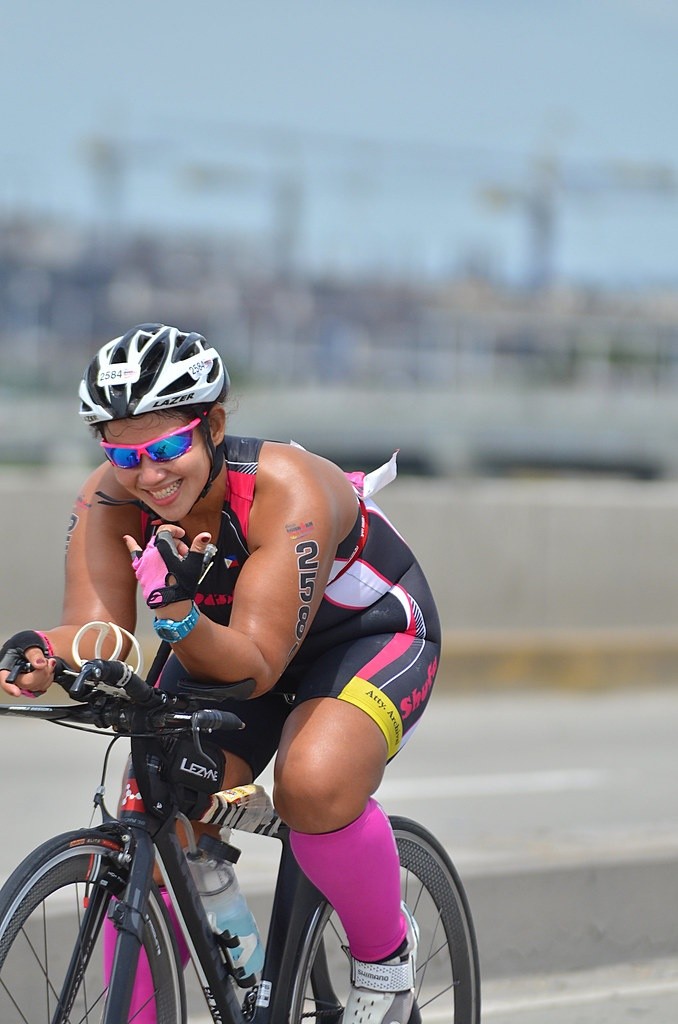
[0,321,443,1023]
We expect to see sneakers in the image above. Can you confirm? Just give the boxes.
[340,901,421,1024]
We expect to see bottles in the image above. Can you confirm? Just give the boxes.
[185,835,267,981]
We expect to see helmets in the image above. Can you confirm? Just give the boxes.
[79,322,231,427]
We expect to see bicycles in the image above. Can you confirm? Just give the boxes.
[0,652,485,1024]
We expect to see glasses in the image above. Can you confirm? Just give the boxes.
[99,411,207,469]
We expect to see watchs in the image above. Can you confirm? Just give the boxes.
[152,600,202,645]
